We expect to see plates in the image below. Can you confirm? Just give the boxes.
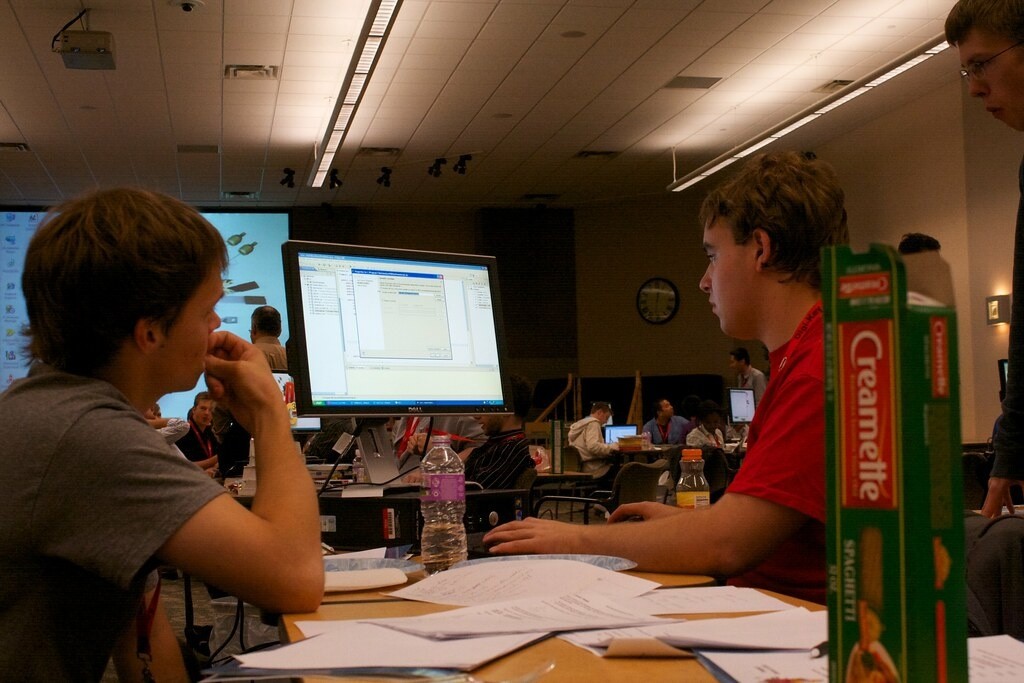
[323,557,425,574]
[448,554,638,572]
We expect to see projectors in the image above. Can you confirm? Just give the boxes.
[59,29,117,70]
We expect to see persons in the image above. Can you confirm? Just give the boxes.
[140,303,533,538]
[641,397,691,487]
[729,348,766,406]
[485,148,851,604]
[0,190,323,683]
[567,403,625,516]
[942,1,1023,518]
[686,400,725,451]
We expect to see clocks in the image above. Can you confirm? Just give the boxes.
[636,277,680,325]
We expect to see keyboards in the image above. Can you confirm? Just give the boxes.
[408,531,505,560]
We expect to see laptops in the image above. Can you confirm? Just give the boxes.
[605,424,638,444]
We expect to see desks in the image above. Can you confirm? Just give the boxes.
[534,470,592,525]
[279,546,828,683]
[605,442,748,465]
[226,476,527,548]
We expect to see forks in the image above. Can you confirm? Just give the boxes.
[403,663,555,683]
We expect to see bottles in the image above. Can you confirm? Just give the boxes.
[675,449,710,510]
[419,436,467,574]
[354,450,367,483]
[639,428,652,450]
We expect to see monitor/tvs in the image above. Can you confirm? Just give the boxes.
[280,240,515,498]
[270,369,320,459]
[998,358,1009,401]
[725,387,756,425]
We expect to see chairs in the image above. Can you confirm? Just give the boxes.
[663,446,736,505]
[555,445,613,520]
[963,514,1024,641]
[532,457,674,524]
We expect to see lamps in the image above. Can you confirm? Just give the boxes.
[280,154,472,188]
[985,294,1011,328]
[665,31,952,192]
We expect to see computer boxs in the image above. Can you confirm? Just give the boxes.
[317,489,532,551]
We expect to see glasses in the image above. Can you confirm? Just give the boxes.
[248,328,253,333]
